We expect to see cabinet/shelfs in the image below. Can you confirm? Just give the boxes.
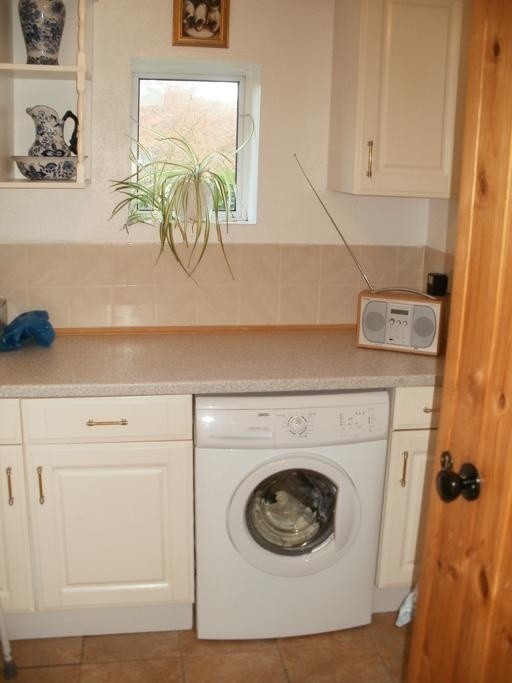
[375,387,442,589]
[328,1,463,199]
[0,396,34,614]
[22,395,196,609]
[0,0,90,189]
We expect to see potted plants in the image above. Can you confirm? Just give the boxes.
[107,111,255,288]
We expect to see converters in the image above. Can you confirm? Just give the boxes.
[426,272,448,296]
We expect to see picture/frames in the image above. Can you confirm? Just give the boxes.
[172,0,231,48]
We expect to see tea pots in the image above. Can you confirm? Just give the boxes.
[24,105,78,157]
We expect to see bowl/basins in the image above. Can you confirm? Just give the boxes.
[10,153,76,180]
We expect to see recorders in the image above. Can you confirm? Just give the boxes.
[354,290,444,357]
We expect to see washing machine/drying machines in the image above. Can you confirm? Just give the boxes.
[192,391,393,643]
